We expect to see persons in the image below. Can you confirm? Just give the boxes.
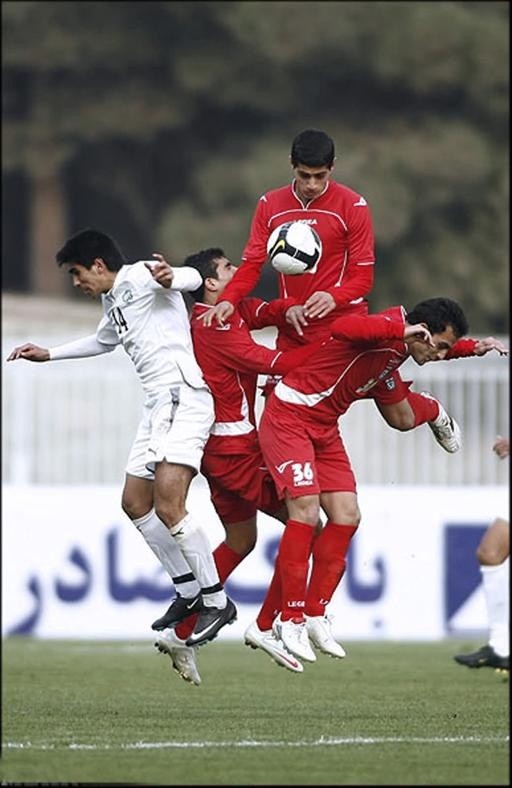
[5,230,237,644]
[159,247,333,685]
[199,127,462,452]
[453,434,509,671]
[243,298,507,662]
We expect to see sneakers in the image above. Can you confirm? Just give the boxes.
[150,590,203,631]
[420,391,462,454]
[153,627,202,687]
[243,610,348,673]
[184,595,238,649]
[452,643,511,679]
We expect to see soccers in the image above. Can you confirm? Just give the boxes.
[266,221,321,275]
